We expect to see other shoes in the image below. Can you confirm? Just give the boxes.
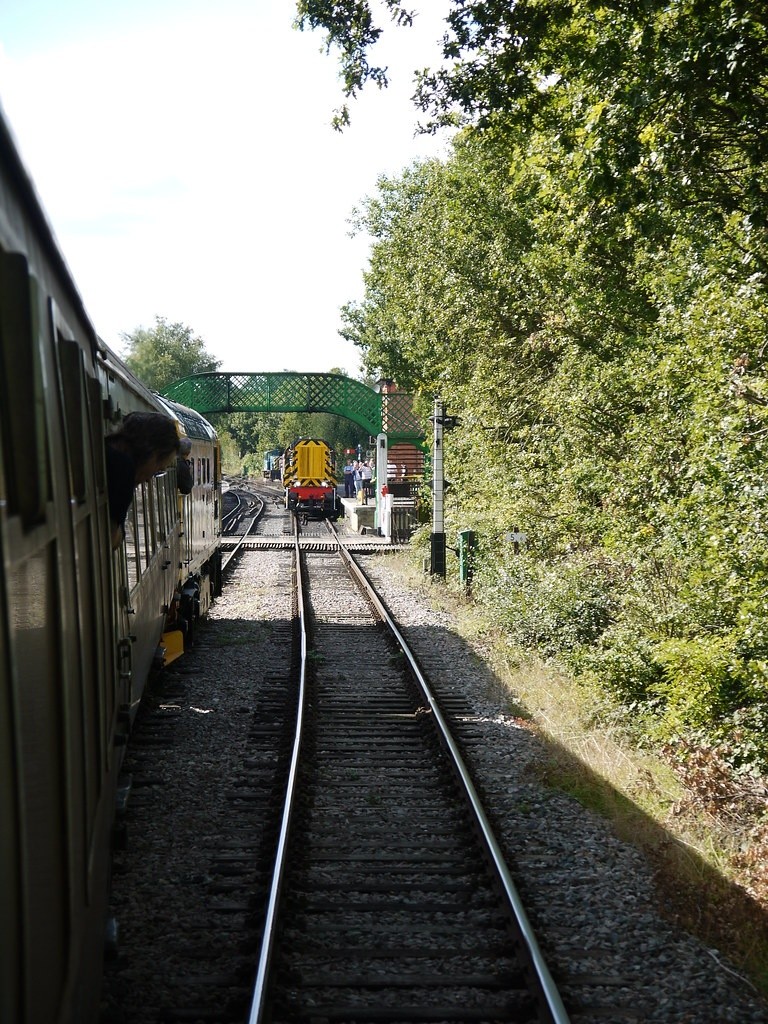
[343,495,349,498]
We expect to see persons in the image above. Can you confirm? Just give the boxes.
[342,458,408,499]
[178,437,194,494]
[104,411,179,551]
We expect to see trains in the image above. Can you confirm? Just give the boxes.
[0,105,223,1024]
[262,438,341,526]
